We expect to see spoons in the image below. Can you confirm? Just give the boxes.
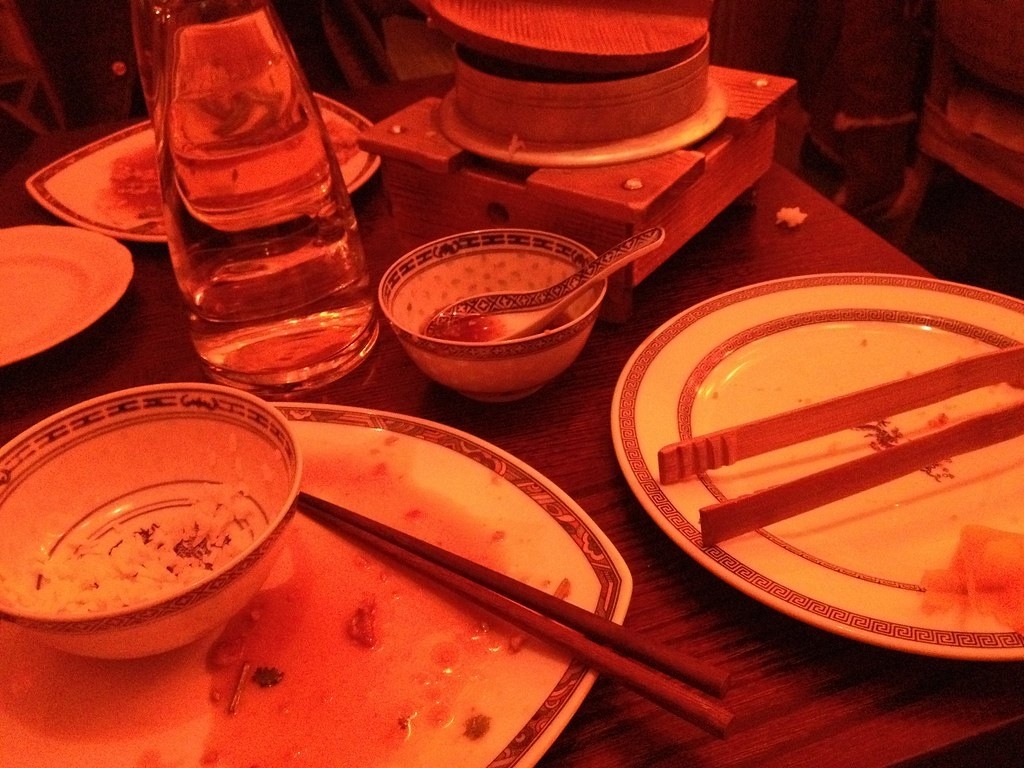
[427,227,666,343]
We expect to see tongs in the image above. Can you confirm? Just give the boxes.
[656,345,1024,532]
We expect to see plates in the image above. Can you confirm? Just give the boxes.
[612,275,1024,662]
[26,90,383,242]
[0,402,633,768]
[0,225,134,367]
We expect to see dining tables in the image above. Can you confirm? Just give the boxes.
[0,68,1024,768]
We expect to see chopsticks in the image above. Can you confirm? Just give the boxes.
[295,492,732,731]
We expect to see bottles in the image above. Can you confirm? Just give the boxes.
[129,0,378,402]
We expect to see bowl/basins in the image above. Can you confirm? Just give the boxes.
[380,228,605,398]
[0,383,300,661]
